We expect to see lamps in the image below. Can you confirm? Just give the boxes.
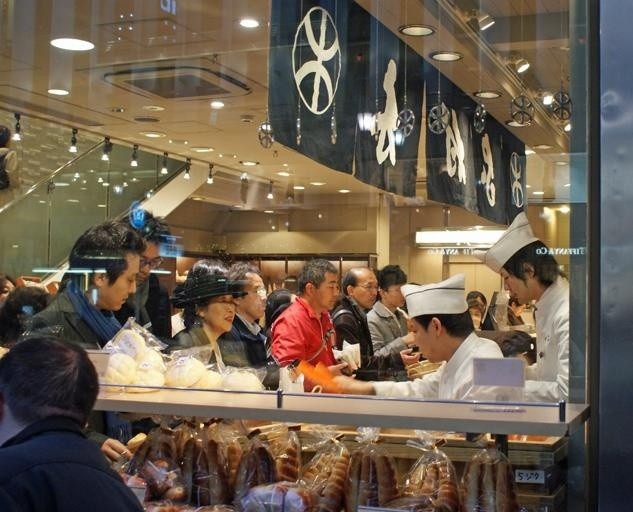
[456,0,570,143]
[9,111,284,199]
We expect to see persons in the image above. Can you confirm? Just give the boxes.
[0,124,24,208]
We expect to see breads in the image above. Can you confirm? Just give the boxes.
[117,426,511,512]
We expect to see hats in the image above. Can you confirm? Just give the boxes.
[399,274,469,319]
[473,212,540,273]
[171,275,249,311]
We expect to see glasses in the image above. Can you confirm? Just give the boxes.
[138,257,164,269]
